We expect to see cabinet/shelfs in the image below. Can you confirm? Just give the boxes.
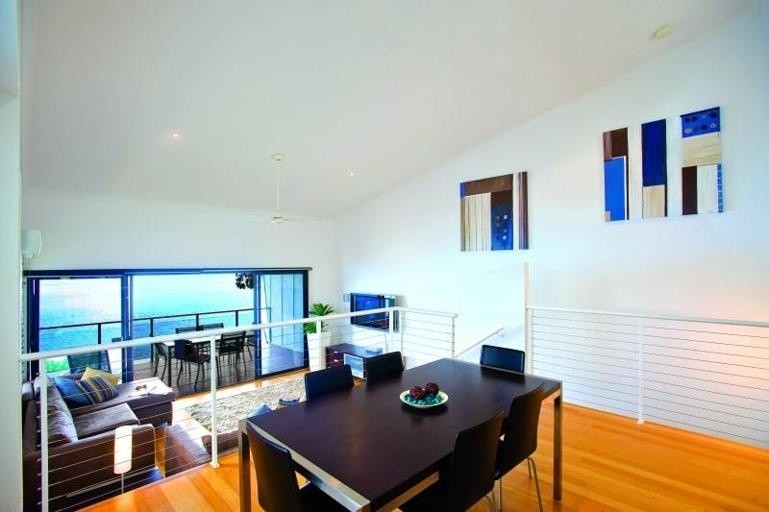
[325,343,382,382]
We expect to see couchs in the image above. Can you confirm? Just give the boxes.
[21,368,175,512]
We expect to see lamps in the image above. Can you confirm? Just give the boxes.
[22,228,41,266]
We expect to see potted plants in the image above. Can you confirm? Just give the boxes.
[299,300,337,372]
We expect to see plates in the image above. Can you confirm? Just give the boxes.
[399,390,449,410]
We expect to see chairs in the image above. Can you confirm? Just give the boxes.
[150,323,260,389]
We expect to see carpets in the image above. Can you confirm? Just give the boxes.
[183,377,307,433]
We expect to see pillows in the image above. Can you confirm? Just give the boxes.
[54,365,120,406]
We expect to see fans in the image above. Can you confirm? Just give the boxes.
[241,153,313,225]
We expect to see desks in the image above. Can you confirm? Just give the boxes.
[239,357,563,512]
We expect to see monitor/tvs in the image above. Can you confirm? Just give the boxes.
[350,292,398,331]
